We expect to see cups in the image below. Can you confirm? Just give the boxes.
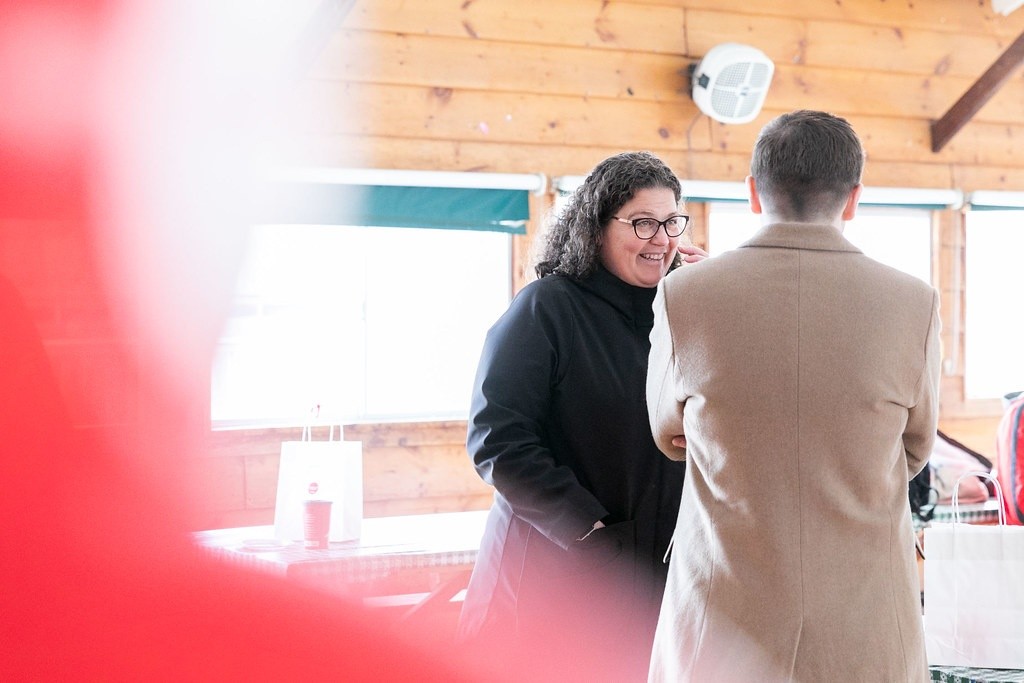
[303,500,334,551]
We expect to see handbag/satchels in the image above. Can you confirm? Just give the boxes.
[273,402,363,541]
[924,470,1024,671]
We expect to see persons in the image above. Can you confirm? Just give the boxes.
[456,151,715,656]
[641,108,946,683]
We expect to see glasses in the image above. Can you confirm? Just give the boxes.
[611,214,689,240]
